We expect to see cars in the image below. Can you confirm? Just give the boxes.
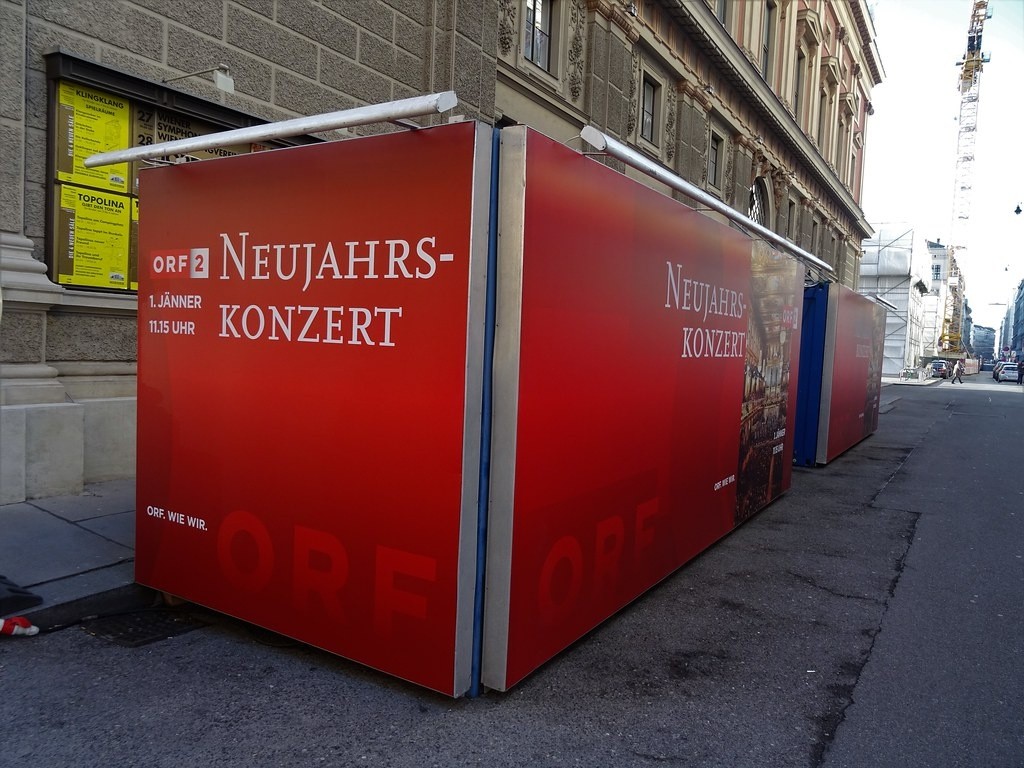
[931,360,953,379]
[992,361,1019,383]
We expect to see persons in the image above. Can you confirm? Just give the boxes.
[1017,360,1024,385]
[951,360,964,384]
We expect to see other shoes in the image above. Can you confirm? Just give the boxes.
[952,383,955,384]
[960,382,964,384]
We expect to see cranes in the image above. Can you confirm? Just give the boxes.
[937,0,991,357]
